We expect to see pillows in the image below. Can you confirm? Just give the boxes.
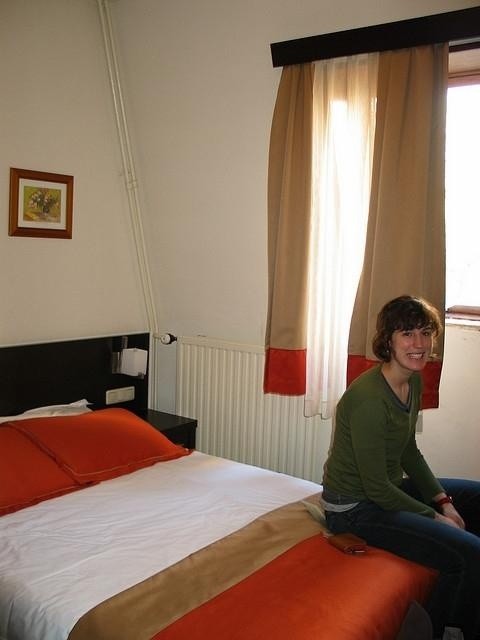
[0,405,193,513]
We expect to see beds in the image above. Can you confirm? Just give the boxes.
[1,400,444,639]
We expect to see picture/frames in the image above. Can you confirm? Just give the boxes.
[8,166,76,241]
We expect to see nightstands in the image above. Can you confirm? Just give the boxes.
[99,408,199,456]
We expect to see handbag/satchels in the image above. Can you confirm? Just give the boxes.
[329,532,367,554]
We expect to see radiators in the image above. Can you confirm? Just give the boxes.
[173,334,318,483]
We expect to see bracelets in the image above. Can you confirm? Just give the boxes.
[436,495,454,507]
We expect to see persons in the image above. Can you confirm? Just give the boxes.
[318,294,480,639]
